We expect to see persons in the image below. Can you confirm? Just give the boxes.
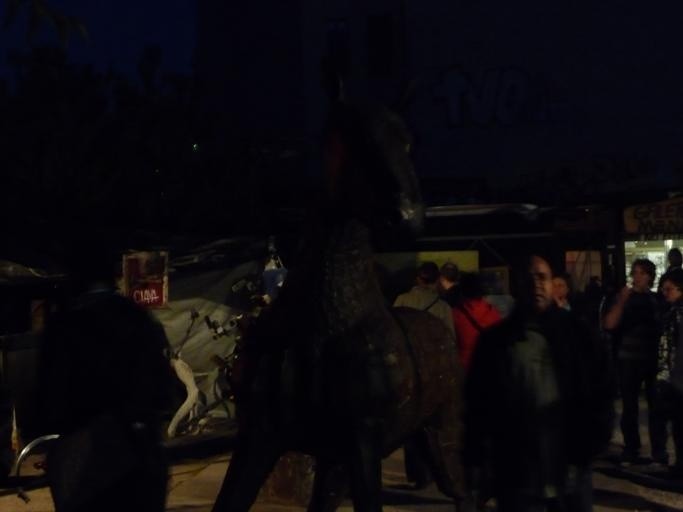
[464,255,614,512]
[24,227,181,511]
[552,257,683,473]
[393,254,503,367]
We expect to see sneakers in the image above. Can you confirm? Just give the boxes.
[616,455,639,468]
[641,462,671,473]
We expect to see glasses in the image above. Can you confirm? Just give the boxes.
[659,285,680,292]
